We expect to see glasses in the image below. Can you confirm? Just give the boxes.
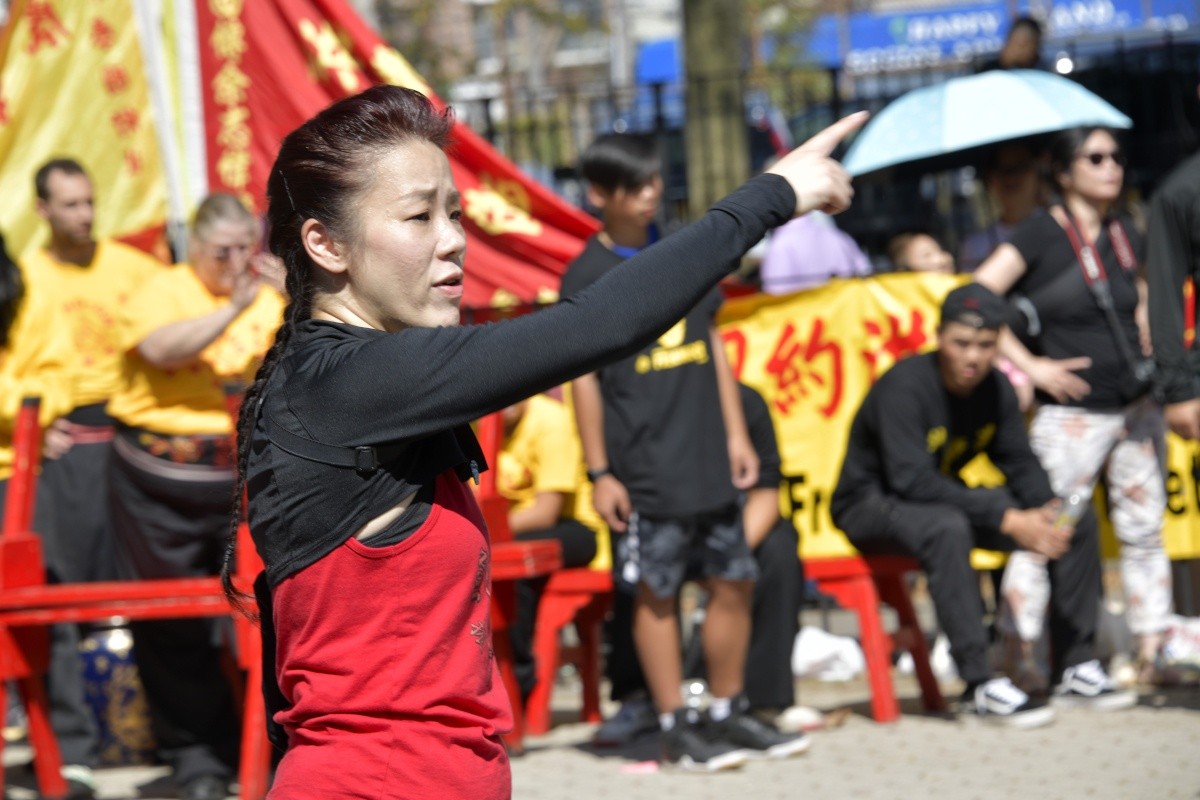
[1078,150,1122,167]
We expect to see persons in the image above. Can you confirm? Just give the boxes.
[968,124,1200,689]
[759,14,1065,300]
[830,283,1138,726]
[464,378,828,744]
[560,129,816,771]
[105,196,287,800]
[1,158,175,793]
[218,85,871,800]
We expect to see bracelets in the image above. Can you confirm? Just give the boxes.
[585,466,613,481]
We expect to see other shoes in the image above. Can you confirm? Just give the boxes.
[594,697,659,748]
[177,773,227,800]
[750,703,825,733]
[1049,658,1136,710]
[956,676,1057,732]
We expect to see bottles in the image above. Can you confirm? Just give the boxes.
[1030,485,1092,566]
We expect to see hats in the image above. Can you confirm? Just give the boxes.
[940,284,1006,331]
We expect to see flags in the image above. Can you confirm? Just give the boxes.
[1,0,184,277]
[177,0,604,312]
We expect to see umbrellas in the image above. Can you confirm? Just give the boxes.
[840,68,1135,182]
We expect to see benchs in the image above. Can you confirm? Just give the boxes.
[1,385,944,800]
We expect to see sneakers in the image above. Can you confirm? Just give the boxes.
[708,698,812,759]
[658,708,746,773]
